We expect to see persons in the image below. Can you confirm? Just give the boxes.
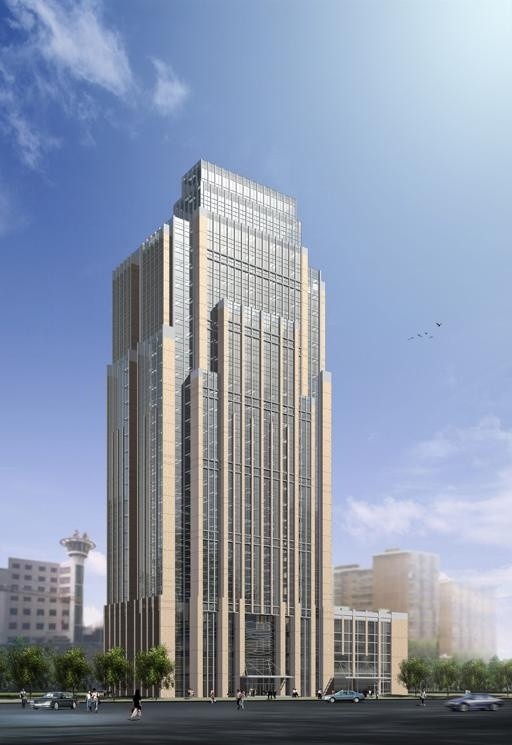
[368,689,372,695]
[190,688,194,695]
[130,690,142,720]
[294,689,298,696]
[292,688,296,698]
[188,687,191,696]
[237,689,242,710]
[90,688,100,713]
[267,690,277,699]
[331,687,344,694]
[317,688,322,699]
[20,688,30,707]
[239,690,246,710]
[418,688,427,706]
[210,690,217,703]
[86,691,93,712]
